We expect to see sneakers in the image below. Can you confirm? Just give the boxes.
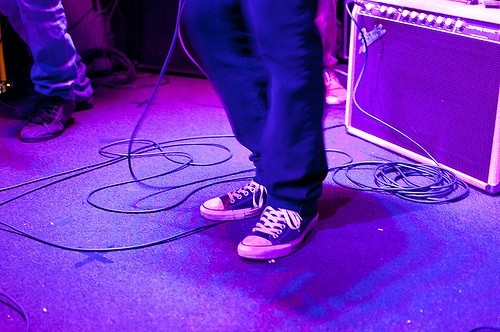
[20,97,75,141]
[323,70,347,104]
[237,203,318,259]
[200,179,269,221]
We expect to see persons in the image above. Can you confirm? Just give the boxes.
[180,0,328,260]
[314,0,347,105]
[0,0,95,143]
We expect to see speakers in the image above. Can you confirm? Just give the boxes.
[345,0,500,195]
